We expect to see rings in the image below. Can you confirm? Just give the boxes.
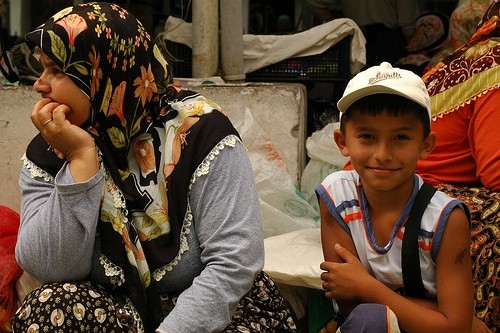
[43,118,52,126]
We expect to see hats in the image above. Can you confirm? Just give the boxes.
[336,62,431,133]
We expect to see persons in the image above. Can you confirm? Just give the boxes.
[341,0,500,333]
[315,61,491,333]
[12,2,299,333]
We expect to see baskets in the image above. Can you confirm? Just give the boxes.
[164,38,192,78]
[246,36,351,81]
